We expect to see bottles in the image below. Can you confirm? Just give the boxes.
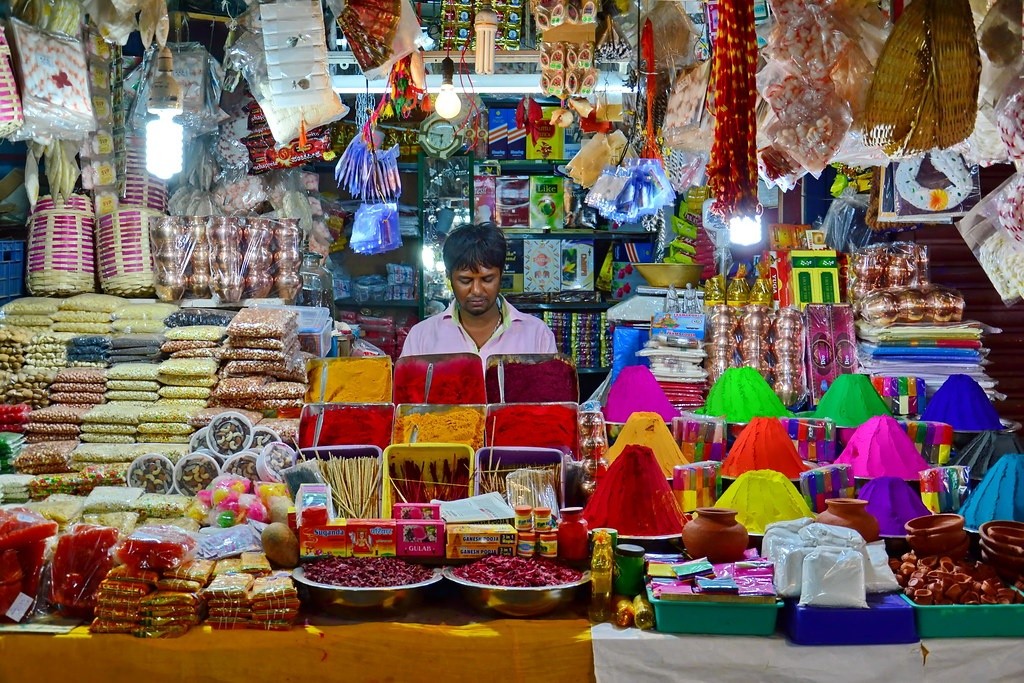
[513,505,653,630]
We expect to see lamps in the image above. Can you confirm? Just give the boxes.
[472,0,501,74]
[143,47,186,179]
[726,214,765,245]
[433,0,461,118]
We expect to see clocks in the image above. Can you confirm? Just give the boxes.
[419,111,468,165]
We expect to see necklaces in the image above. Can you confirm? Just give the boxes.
[477,313,501,352]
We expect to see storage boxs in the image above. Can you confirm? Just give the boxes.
[900,585,1024,636]
[277,303,332,357]
[644,575,786,637]
[784,595,919,645]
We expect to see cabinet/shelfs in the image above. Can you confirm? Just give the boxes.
[315,151,420,323]
[439,155,661,376]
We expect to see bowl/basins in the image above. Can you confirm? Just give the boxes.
[438,560,594,619]
[290,558,444,622]
[126,411,299,498]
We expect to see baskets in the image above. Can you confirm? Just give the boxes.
[863,1,982,159]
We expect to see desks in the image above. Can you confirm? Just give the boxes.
[585,623,1024,683]
[0,615,592,683]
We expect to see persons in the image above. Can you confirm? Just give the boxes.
[400,221,558,376]
